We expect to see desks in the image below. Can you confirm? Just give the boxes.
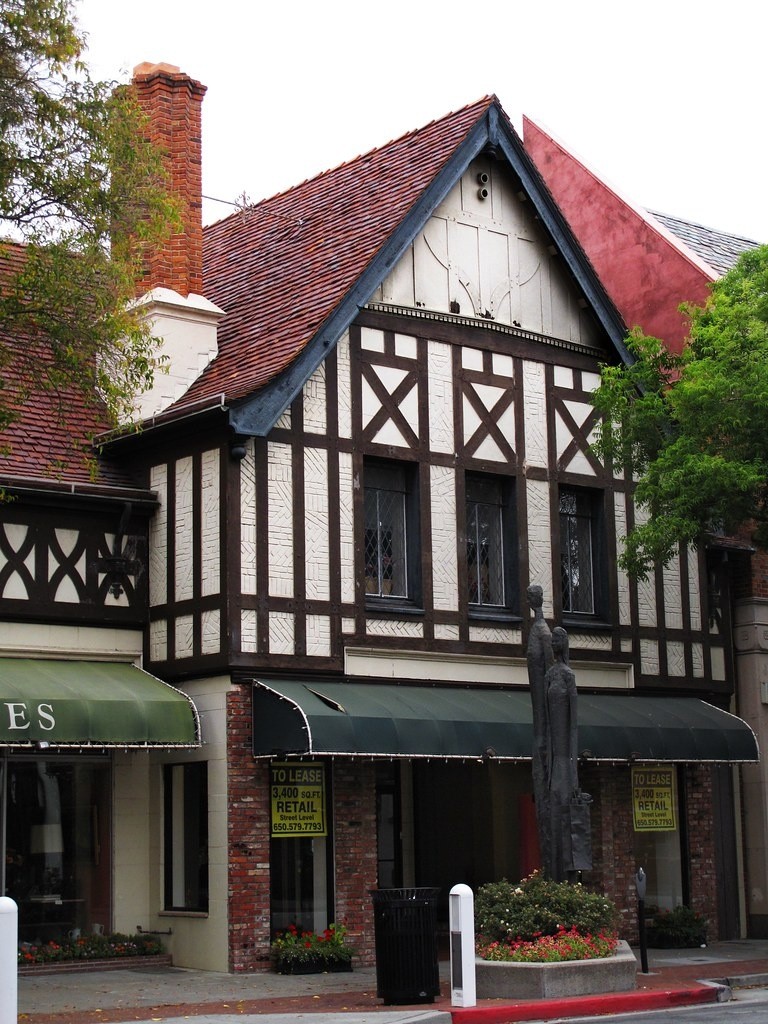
[21,899,86,936]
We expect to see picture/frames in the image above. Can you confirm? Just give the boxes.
[62,804,100,867]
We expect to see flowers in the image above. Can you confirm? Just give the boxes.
[6,849,23,868]
[324,923,355,959]
[365,552,392,575]
[17,932,162,964]
[468,574,484,591]
[301,931,324,962]
[473,867,622,962]
[270,925,300,961]
[656,905,706,934]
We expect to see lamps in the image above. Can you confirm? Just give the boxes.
[478,753,486,765]
[578,749,592,761]
[29,823,64,901]
[627,752,641,761]
[481,746,498,761]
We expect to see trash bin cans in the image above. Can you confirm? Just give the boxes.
[366,887,441,1006]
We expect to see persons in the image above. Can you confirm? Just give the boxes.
[528,584,593,884]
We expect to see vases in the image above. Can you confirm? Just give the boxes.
[329,952,353,973]
[281,957,299,974]
[6,867,15,894]
[365,577,393,595]
[647,928,701,949]
[476,941,638,999]
[468,590,490,602]
[306,957,326,973]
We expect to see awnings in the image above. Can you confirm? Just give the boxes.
[0,658,202,749]
[252,678,760,763]
